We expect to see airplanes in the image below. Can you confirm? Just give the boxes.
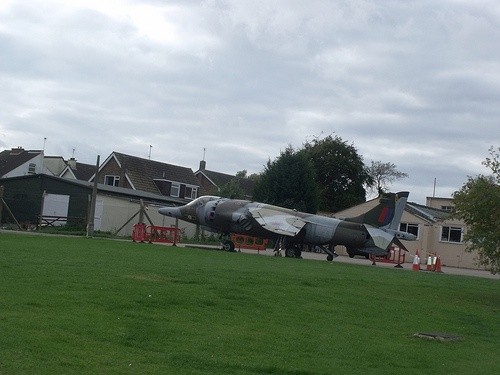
[156,186,417,261]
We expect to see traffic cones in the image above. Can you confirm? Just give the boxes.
[432,252,437,271]
[425,251,433,270]
[411,249,420,270]
[434,255,442,272]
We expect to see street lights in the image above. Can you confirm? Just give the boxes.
[71,148,76,158]
[44,137,48,154]
[149,144,152,158]
